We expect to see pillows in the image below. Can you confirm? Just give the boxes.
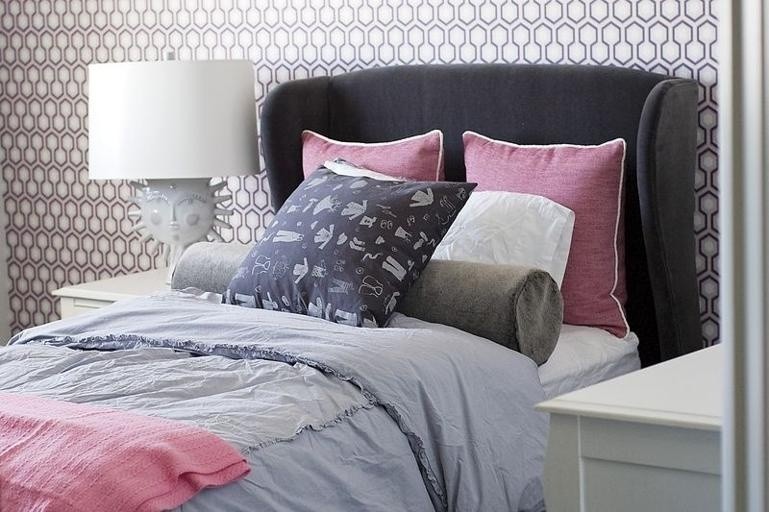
[170,242,564,366]
[324,159,576,292]
[301,130,445,181]
[221,156,479,328]
[463,131,631,342]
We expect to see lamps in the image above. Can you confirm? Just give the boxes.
[85,58,261,282]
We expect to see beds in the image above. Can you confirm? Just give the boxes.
[0,63,705,512]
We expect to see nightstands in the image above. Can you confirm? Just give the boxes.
[534,341,725,510]
